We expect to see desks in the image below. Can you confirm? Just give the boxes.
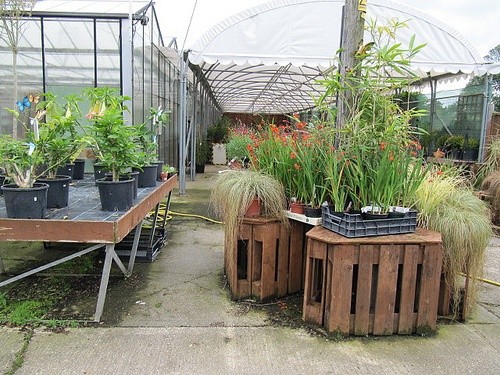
[0,171,179,325]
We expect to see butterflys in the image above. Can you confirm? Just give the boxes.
[16,96,31,112]
[29,93,40,103]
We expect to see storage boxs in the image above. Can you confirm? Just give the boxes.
[320,204,419,238]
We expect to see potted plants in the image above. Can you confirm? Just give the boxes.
[207,15,480,239]
[0,85,174,220]
